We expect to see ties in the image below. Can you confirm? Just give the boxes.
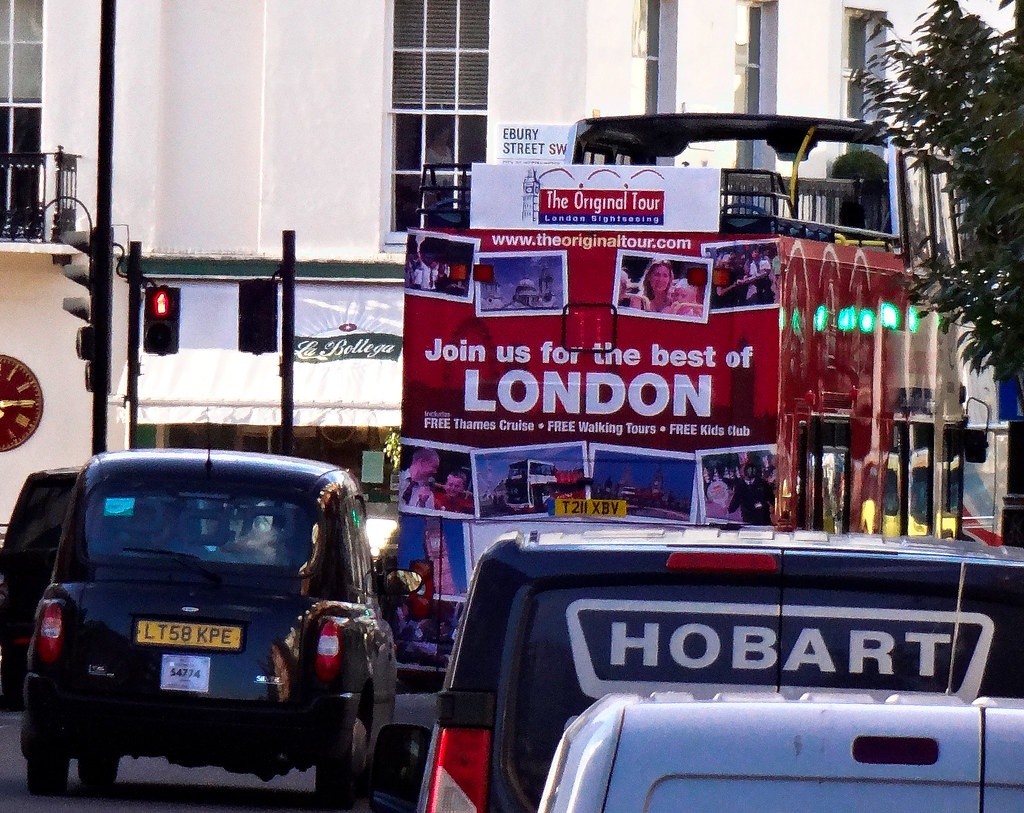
[429,269,433,288]
[403,477,419,505]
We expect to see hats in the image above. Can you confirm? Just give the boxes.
[515,279,537,296]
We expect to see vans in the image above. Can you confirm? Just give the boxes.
[520,680,1024,813]
[373,519,1024,812]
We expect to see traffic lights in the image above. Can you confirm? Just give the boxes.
[145,282,178,354]
[771,289,920,337]
[57,224,99,390]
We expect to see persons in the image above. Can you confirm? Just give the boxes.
[413,253,447,292]
[400,450,440,510]
[727,463,775,526]
[716,248,776,307]
[642,260,674,313]
[433,469,474,513]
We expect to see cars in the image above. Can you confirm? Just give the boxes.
[0,451,425,807]
